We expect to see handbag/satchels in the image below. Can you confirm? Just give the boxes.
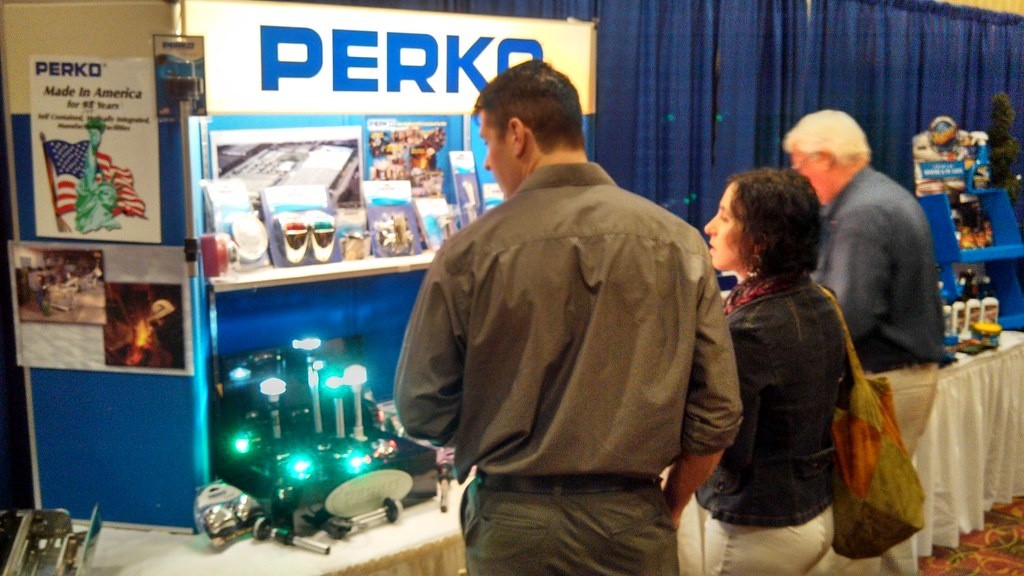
[808,280,926,558]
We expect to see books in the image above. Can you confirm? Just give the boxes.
[415,198,453,250]
[259,184,343,268]
[449,150,504,227]
[363,180,421,257]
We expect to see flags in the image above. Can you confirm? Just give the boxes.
[44,139,144,218]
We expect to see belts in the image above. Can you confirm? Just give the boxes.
[475,467,660,494]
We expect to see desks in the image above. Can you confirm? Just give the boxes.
[907,329,1024,555]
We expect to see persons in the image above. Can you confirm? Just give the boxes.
[74,127,127,233]
[693,170,845,576]
[783,110,945,576]
[35,278,51,316]
[150,301,182,358]
[393,60,743,576]
